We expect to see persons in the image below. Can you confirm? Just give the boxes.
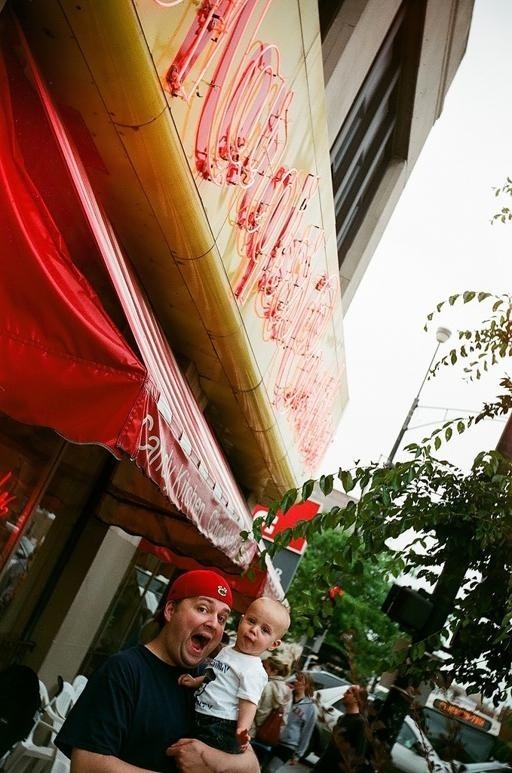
[261,669,319,772]
[51,567,263,772]
[164,595,291,753]
[246,656,293,773]
[310,684,370,772]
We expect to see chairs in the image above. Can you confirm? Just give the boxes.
[0,675,89,771]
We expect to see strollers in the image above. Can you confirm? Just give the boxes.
[0,663,65,762]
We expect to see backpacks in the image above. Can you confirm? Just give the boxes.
[254,706,285,748]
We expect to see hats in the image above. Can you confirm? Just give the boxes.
[165,569,234,611]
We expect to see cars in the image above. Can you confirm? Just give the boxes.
[1,520,36,609]
[281,639,511,773]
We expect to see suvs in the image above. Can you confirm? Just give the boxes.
[114,565,171,637]
